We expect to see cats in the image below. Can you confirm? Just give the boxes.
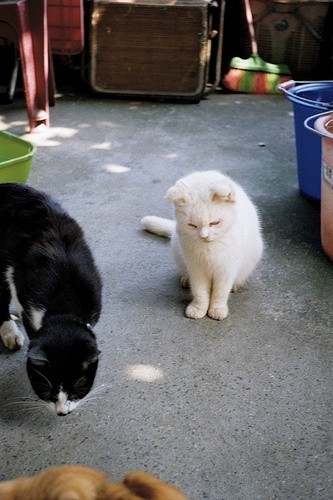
[141,170,264,320]
[0,464,193,500]
[0,181,103,417]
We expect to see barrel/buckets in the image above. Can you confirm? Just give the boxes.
[276,80,333,200]
[304,110,333,262]
[0,130,36,184]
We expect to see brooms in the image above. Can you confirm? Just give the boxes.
[220,0,293,96]
